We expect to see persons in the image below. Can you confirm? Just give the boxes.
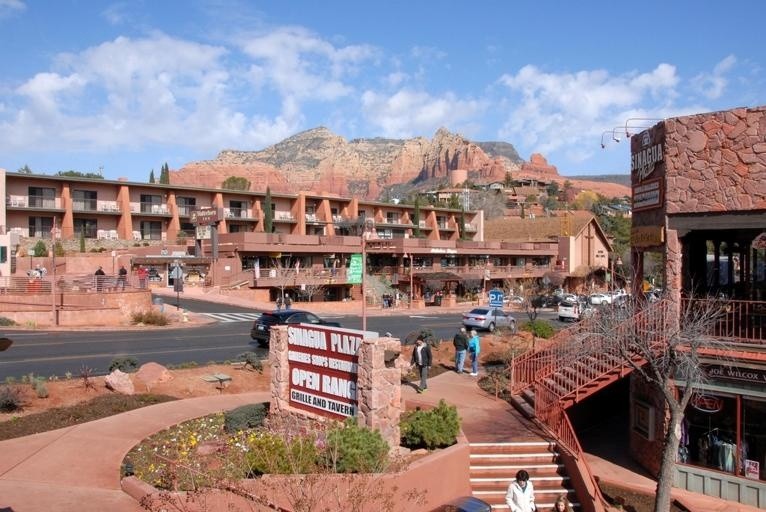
[382,289,402,308]
[34,263,47,279]
[275,293,282,310]
[504,470,536,512]
[550,495,574,512]
[113,266,127,291]
[453,328,469,373]
[410,334,432,393]
[137,265,149,288]
[467,329,480,376]
[284,293,292,310]
[95,266,105,291]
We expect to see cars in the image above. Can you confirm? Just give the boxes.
[502,288,664,308]
[462,307,516,332]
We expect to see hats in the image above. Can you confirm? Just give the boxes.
[417,335,423,341]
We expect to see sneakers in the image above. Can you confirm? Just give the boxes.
[470,373,478,377]
[417,388,423,393]
[422,388,428,391]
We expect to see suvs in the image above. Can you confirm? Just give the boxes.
[250,308,344,349]
[556,301,597,323]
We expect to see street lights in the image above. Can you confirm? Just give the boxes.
[482,259,490,291]
[401,249,414,308]
[49,215,62,326]
[360,224,381,332]
[559,256,568,289]
[610,254,623,327]
